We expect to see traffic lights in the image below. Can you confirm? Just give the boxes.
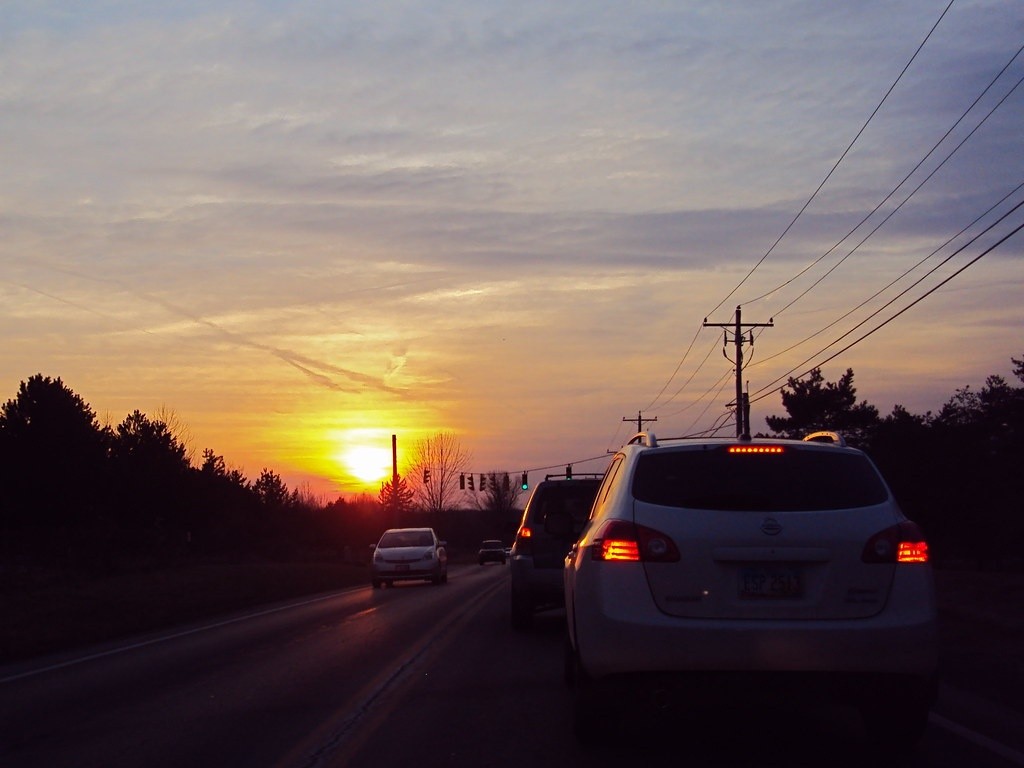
[566,467,572,480]
[522,474,528,490]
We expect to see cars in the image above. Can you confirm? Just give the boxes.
[506,473,607,635]
[477,540,507,565]
[504,548,511,556]
[369,528,448,590]
[565,430,940,748]
